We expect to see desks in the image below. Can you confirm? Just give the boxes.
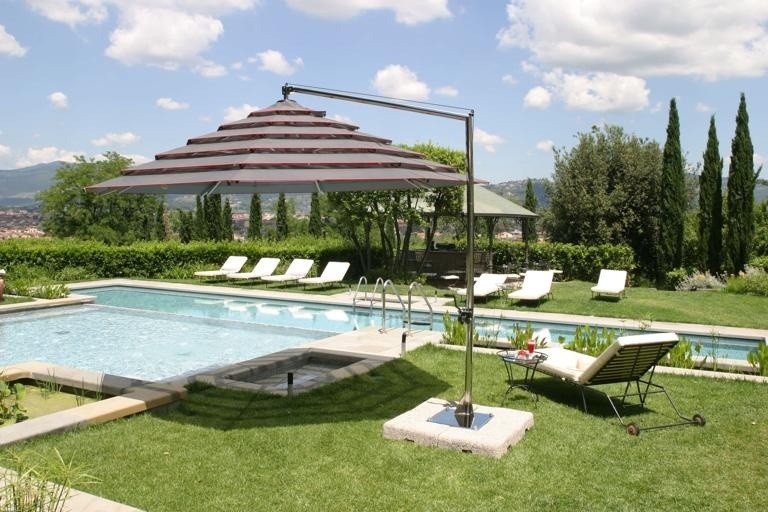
[496,350,548,408]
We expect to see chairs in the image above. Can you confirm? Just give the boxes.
[515,332,706,436]
[194,255,351,292]
[455,270,554,308]
[591,269,628,300]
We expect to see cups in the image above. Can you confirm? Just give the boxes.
[527,339,536,354]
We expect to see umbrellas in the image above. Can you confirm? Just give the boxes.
[367,183,539,218]
[82,84,476,427]
